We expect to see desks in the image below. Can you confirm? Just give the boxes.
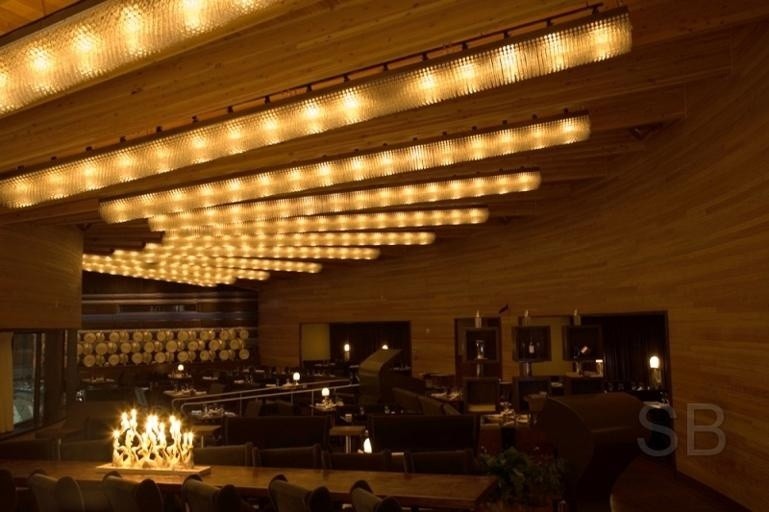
[309,400,345,426]
[163,390,206,401]
[190,409,238,444]
[329,425,368,453]
[2,456,499,510]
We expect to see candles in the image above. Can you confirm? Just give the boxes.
[573,308,581,326]
[474,310,483,329]
[522,310,531,327]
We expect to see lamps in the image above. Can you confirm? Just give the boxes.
[108,405,200,475]
[595,358,604,375]
[315,387,344,409]
[649,352,662,384]
[202,401,225,416]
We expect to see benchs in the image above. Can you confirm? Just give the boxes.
[244,413,337,449]
[371,411,482,471]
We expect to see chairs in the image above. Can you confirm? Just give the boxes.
[347,479,403,511]
[466,376,500,419]
[392,386,465,418]
[194,441,416,476]
[181,476,246,510]
[266,474,331,510]
[100,469,162,512]
[223,398,294,441]
[1,437,117,461]
[24,468,87,512]
